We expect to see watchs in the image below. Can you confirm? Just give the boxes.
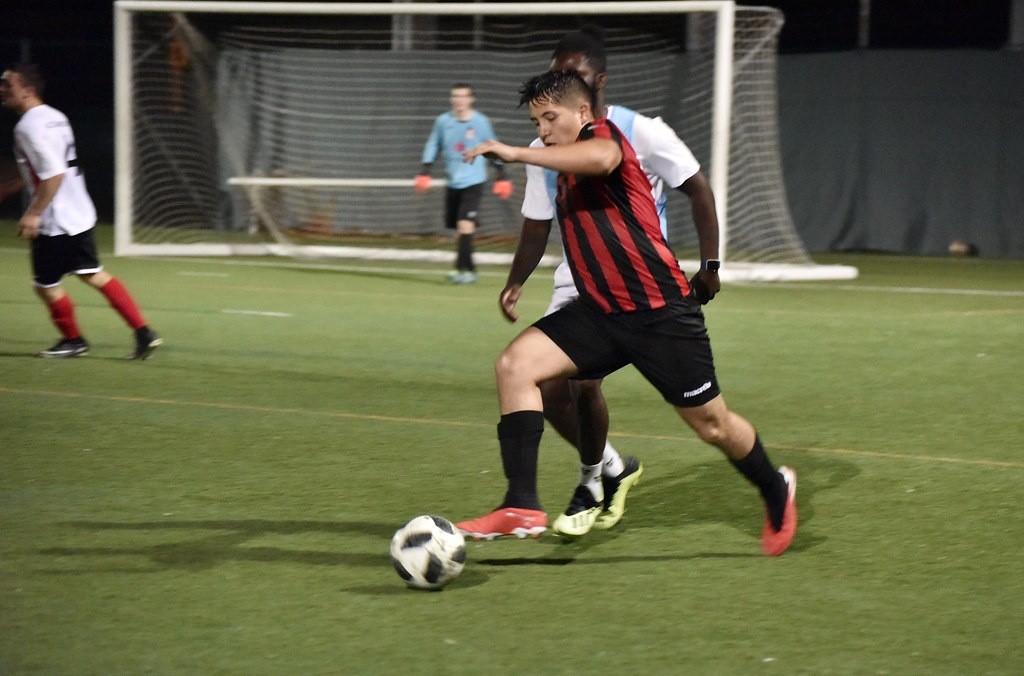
[701,259,720,271]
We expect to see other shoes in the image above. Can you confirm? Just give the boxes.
[452,270,477,283]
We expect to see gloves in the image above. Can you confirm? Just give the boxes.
[493,178,512,198]
[416,174,431,191]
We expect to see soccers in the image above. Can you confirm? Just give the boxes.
[388,514,468,591]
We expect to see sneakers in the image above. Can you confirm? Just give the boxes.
[552,486,605,536]
[761,466,797,557]
[133,331,163,360]
[454,507,547,541]
[39,338,89,357]
[591,455,643,529]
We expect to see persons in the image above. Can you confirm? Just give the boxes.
[416,83,512,282]
[499,34,722,536]
[455,67,799,558]
[0,61,162,358]
